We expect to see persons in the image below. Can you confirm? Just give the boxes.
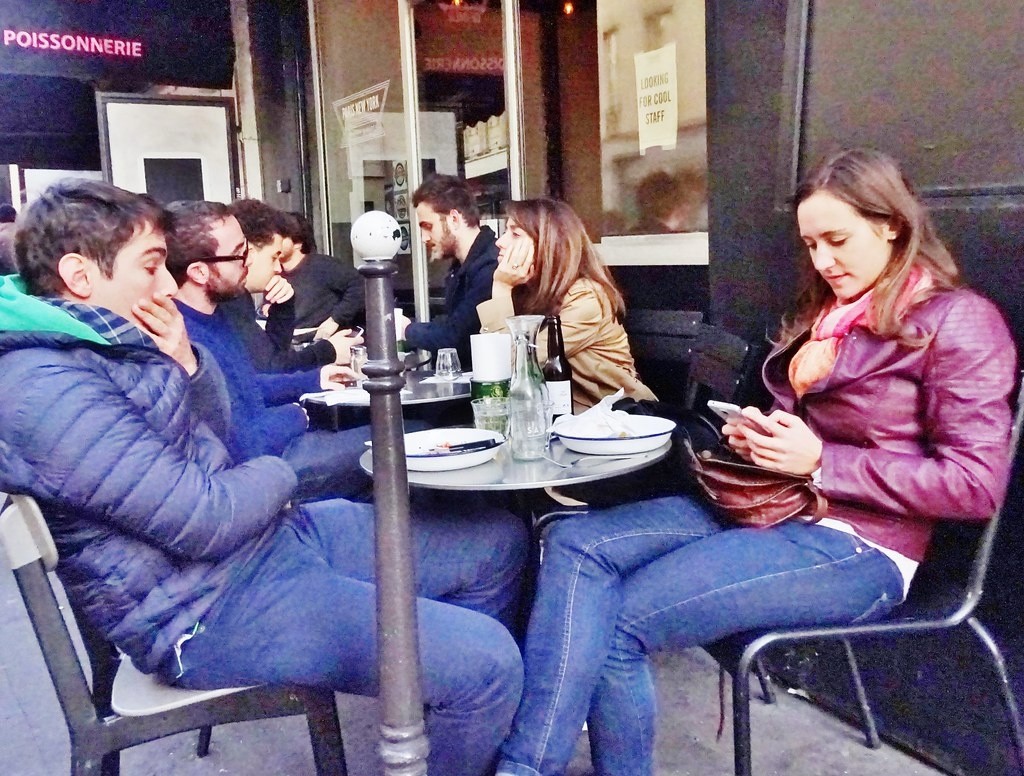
[0,177,527,776]
[164,200,430,502]
[400,172,501,372]
[492,145,1017,776]
[218,198,365,376]
[0,205,20,275]
[262,211,365,341]
[477,198,660,416]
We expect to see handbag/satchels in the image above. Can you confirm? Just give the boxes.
[692,448,814,529]
[546,314,576,426]
[584,397,724,508]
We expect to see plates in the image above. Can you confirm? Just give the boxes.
[551,414,677,454]
[397,428,505,472]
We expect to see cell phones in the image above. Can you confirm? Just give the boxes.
[346,326,365,340]
[708,401,769,437]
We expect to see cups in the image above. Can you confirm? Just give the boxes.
[436,348,461,378]
[472,397,511,438]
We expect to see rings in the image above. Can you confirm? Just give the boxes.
[512,263,518,270]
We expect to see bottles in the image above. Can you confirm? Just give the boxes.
[540,315,574,423]
[348,345,369,379]
[505,315,550,460]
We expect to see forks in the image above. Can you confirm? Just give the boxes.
[544,451,643,469]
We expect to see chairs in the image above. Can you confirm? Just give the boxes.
[700,357,1023,776]
[0,489,349,776]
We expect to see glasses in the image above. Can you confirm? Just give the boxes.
[188,238,249,268]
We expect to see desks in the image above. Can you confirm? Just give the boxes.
[359,410,671,582]
[305,374,471,429]
